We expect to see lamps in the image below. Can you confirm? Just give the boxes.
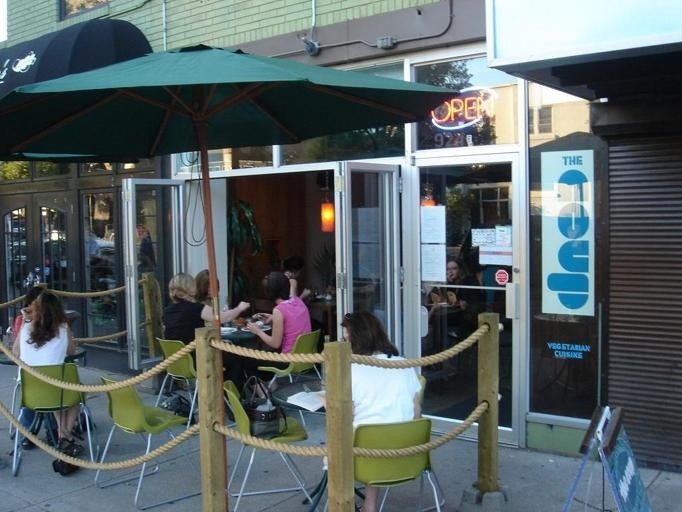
[320,179,335,234]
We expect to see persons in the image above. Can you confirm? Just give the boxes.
[161,253,514,417]
[12,291,80,445]
[14,286,61,451]
[137,225,157,275]
[317,313,424,511]
[84,232,103,267]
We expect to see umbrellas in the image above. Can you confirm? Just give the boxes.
[0,44,461,340]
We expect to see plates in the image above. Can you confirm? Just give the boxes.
[241,324,272,333]
[220,326,238,335]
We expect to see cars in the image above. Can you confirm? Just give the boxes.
[10,238,157,301]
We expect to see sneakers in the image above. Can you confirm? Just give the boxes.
[56,431,84,455]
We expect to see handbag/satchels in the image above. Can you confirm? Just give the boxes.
[242,398,281,439]
[160,392,195,425]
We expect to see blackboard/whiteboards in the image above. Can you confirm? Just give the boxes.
[599,419,650,512]
